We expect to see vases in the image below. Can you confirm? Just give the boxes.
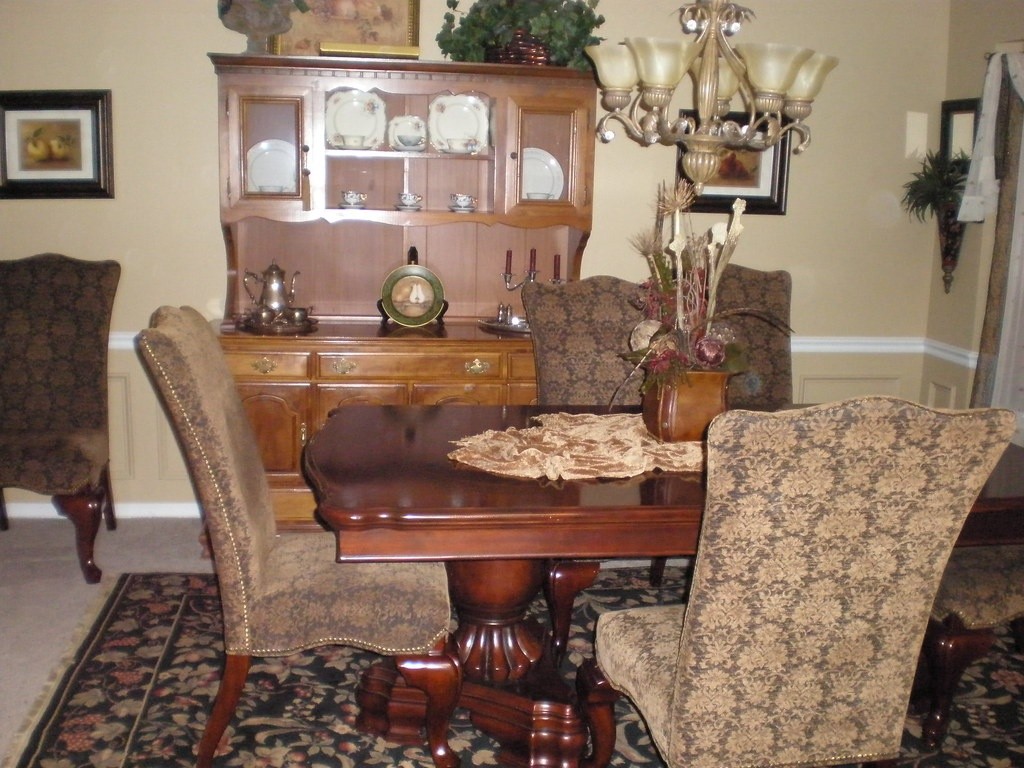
[641,370,735,442]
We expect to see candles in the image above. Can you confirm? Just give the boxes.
[553,255,561,281]
[529,248,536,274]
[505,249,512,274]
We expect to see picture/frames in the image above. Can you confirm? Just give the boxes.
[672,110,793,214]
[266,0,420,63]
[0,89,115,200]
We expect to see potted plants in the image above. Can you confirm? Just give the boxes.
[903,152,970,293]
[437,0,605,72]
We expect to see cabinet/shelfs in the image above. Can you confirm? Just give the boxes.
[209,51,597,325]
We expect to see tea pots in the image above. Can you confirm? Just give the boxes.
[242,262,300,318]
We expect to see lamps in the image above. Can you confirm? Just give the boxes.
[584,1,839,194]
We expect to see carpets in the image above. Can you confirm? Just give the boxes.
[0,568,1024,768]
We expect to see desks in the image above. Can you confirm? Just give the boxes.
[302,403,1024,768]
[202,321,724,528]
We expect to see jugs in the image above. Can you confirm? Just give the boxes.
[285,307,312,322]
[250,304,283,325]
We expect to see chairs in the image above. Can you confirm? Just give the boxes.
[135,305,461,768]
[0,254,120,586]
[523,263,1023,768]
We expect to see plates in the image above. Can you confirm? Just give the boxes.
[246,140,295,193]
[521,147,564,200]
[392,146,426,152]
[449,207,476,214]
[327,91,386,151]
[340,204,365,209]
[439,149,476,154]
[381,264,444,327]
[388,115,426,151]
[428,94,489,154]
[336,145,372,150]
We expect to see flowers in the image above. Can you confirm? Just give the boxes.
[606,179,796,410]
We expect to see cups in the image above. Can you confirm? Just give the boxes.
[526,192,553,200]
[399,193,422,206]
[260,186,283,192]
[342,135,365,146]
[451,194,476,208]
[398,135,424,147]
[447,138,476,149]
[343,192,367,205]
